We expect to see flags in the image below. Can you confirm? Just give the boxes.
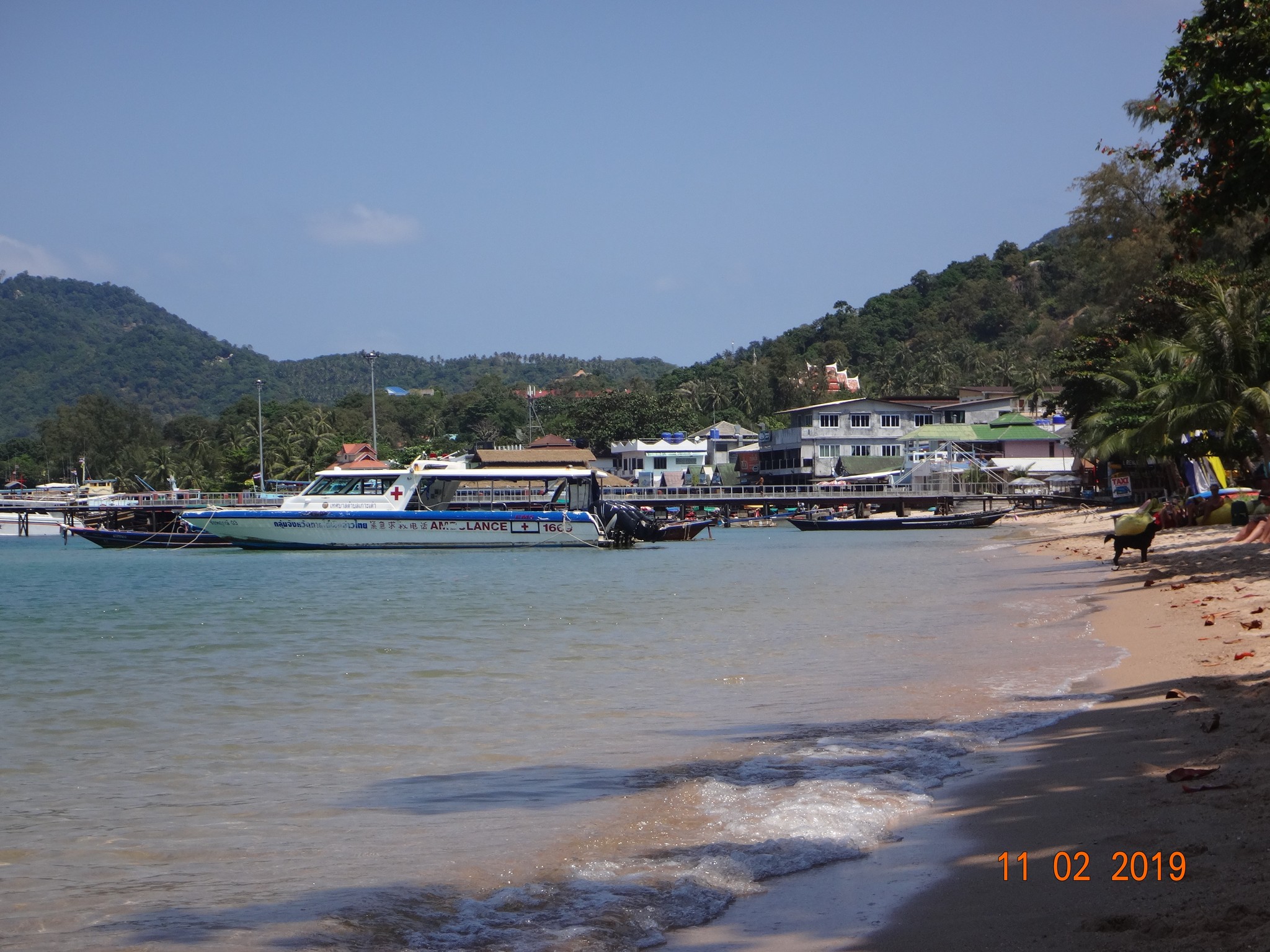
[252,472,261,479]
[244,478,253,485]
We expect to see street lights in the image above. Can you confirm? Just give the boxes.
[252,378,266,491]
[363,352,380,459]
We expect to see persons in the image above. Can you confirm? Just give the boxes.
[939,505,946,512]
[71,468,77,484]
[980,455,987,463]
[1153,484,1224,528]
[1224,489,1270,547]
[756,477,766,498]
[811,505,818,514]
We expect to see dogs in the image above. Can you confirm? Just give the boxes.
[1103,520,1161,567]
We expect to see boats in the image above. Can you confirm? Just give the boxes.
[54,458,712,553]
[784,504,1015,532]
[0,453,139,538]
